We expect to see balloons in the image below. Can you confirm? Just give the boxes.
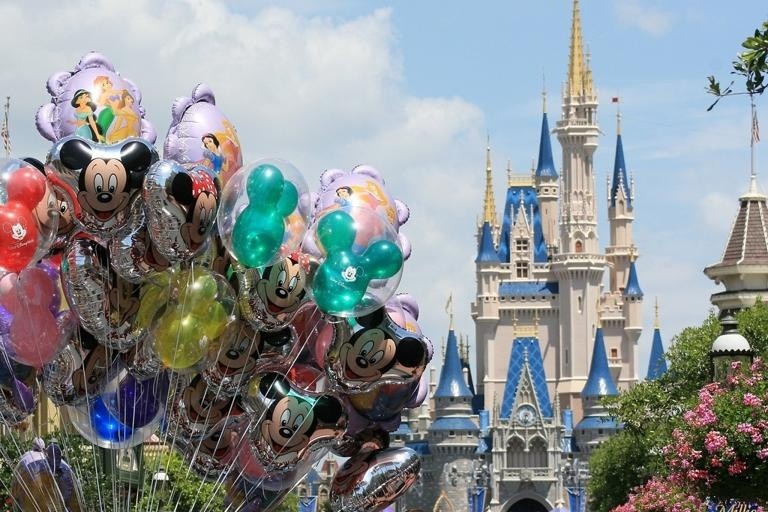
[0,51,434,512]
